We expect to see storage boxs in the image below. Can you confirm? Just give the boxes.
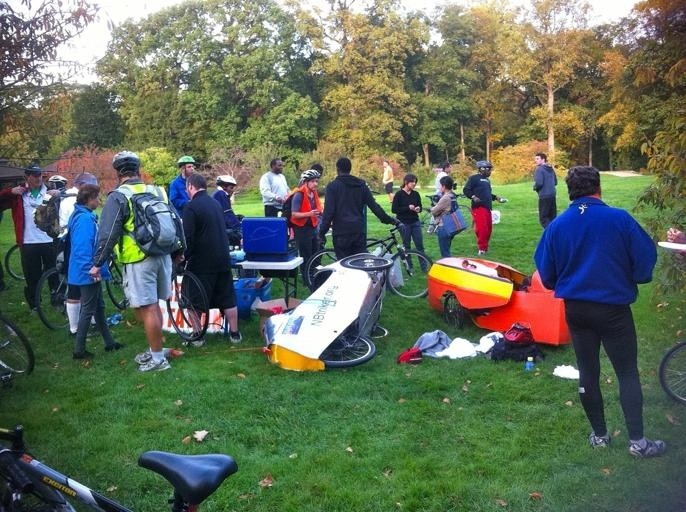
[254,297,303,337]
[233,278,273,318]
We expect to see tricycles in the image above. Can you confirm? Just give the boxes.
[258,249,395,379]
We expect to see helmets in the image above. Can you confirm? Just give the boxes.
[216,174,236,187]
[112,150,140,169]
[177,155,195,168]
[477,160,493,168]
[300,169,321,182]
[48,174,68,184]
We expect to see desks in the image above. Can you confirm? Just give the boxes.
[223,255,305,310]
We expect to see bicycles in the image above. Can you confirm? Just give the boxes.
[169,261,209,341]
[363,232,392,259]
[6,240,44,281]
[303,218,435,298]
[0,313,37,392]
[36,241,130,332]
[0,416,237,512]
[321,222,337,262]
[660,339,686,404]
[425,191,475,233]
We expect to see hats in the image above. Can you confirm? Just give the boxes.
[25,165,42,176]
[76,171,97,185]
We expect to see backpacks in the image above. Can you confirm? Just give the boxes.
[281,192,296,227]
[108,184,187,255]
[33,188,78,238]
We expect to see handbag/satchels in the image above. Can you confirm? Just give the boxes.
[492,340,544,363]
[442,208,467,238]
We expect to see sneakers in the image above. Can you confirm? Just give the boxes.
[73,350,94,360]
[629,438,666,458]
[135,349,170,364]
[427,226,434,234]
[182,331,206,347]
[589,430,611,448]
[104,342,123,351]
[229,327,243,344]
[138,358,171,372]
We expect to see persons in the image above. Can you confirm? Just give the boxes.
[426,162,452,233]
[463,160,505,254]
[390,174,427,275]
[533,165,667,459]
[533,152,558,229]
[666,228,686,257]
[382,161,394,203]
[319,157,400,260]
[430,175,458,258]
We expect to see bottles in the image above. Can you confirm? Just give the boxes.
[525,356,536,370]
[230,238,243,267]
[427,221,437,233]
[371,244,384,256]
[107,314,124,327]
